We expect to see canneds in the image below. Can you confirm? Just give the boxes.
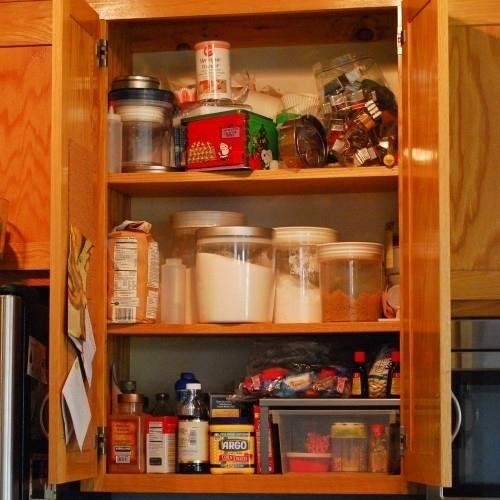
[207,394,256,475]
[195,40,231,103]
[180,108,329,173]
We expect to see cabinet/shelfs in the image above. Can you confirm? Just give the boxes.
[51,1,448,496]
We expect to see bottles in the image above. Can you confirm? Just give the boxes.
[158,209,386,325]
[387,351,401,398]
[367,423,389,473]
[108,87,178,175]
[106,99,124,173]
[350,350,369,398]
[106,371,209,474]
